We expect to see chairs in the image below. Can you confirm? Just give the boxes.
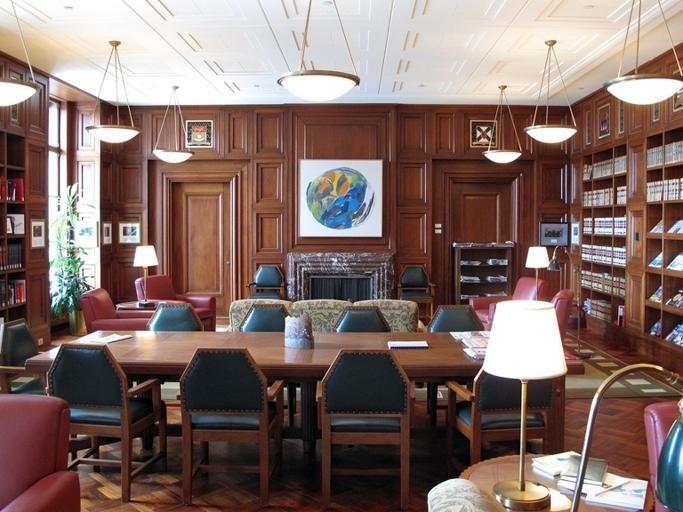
[447,363,560,473]
[315,347,416,510]
[397,264,436,323]
[239,302,298,429]
[246,263,286,299]
[46,343,167,502]
[644,401,683,512]
[427,306,486,426]
[176,347,284,507]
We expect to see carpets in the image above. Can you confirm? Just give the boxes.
[562,329,683,398]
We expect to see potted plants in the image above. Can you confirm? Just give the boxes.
[46,182,96,336]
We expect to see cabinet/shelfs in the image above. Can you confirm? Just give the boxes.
[0,128,52,353]
[452,246,515,305]
[579,126,683,373]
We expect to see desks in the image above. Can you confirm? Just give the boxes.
[25,330,585,475]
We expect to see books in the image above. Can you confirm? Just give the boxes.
[103,334,132,343]
[582,155,627,328]
[388,340,429,350]
[452,241,515,300]
[0,176,27,308]
[647,141,683,346]
[531,450,649,512]
[450,331,490,359]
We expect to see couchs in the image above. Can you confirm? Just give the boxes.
[81,277,573,334]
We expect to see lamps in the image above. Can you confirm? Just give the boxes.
[276,0,361,103]
[482,0,683,164]
[132,246,159,306]
[547,245,591,359]
[483,300,568,511]
[525,247,550,299]
[0,0,194,165]
[570,363,683,512]
